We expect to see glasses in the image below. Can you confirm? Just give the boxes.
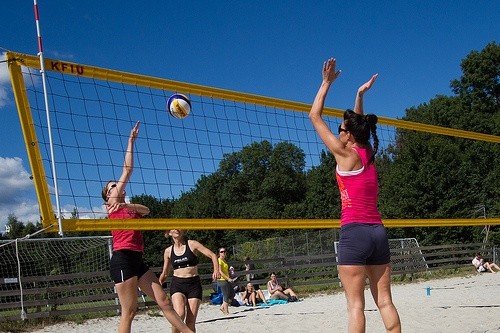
[338,125,352,134]
[107,183,118,194]
[220,251,226,253]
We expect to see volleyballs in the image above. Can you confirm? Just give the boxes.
[166,93,192,119]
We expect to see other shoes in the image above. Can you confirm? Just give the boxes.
[288,296,296,302]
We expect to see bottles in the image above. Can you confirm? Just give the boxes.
[426,284,430,297]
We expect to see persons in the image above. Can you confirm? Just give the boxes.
[242,282,268,307]
[472,252,500,273]
[216,248,233,315]
[159,229,221,333]
[309,58,401,333]
[267,272,301,301]
[243,255,259,290]
[102,119,195,333]
